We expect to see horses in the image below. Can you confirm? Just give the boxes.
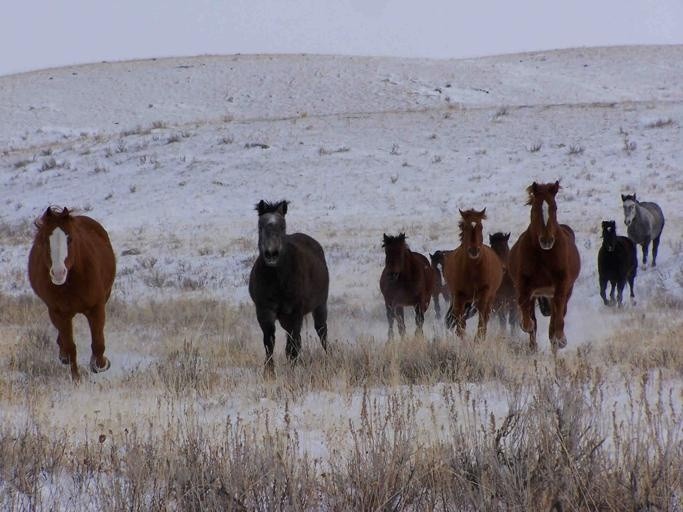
[617,193,665,269]
[594,218,639,310]
[378,176,583,357]
[248,198,332,381]
[27,204,118,381]
